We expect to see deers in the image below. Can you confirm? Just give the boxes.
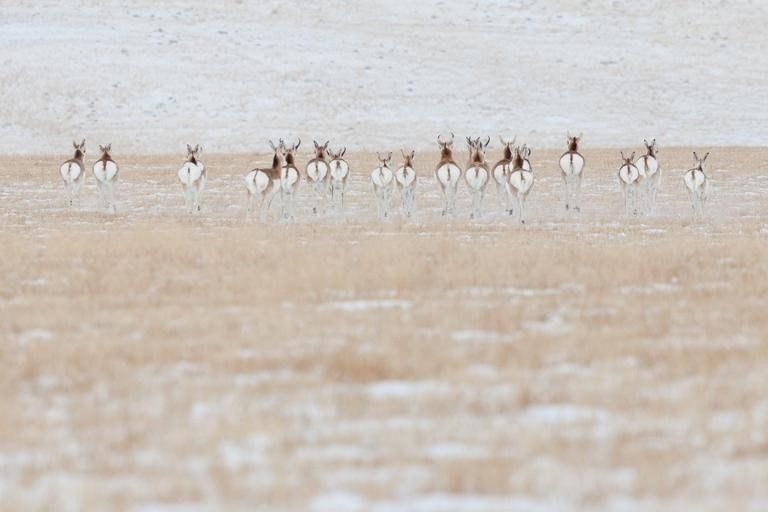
[59,138,88,208]
[616,138,662,215]
[91,143,121,210]
[682,150,712,230]
[557,128,586,213]
[176,141,208,216]
[242,131,534,224]
